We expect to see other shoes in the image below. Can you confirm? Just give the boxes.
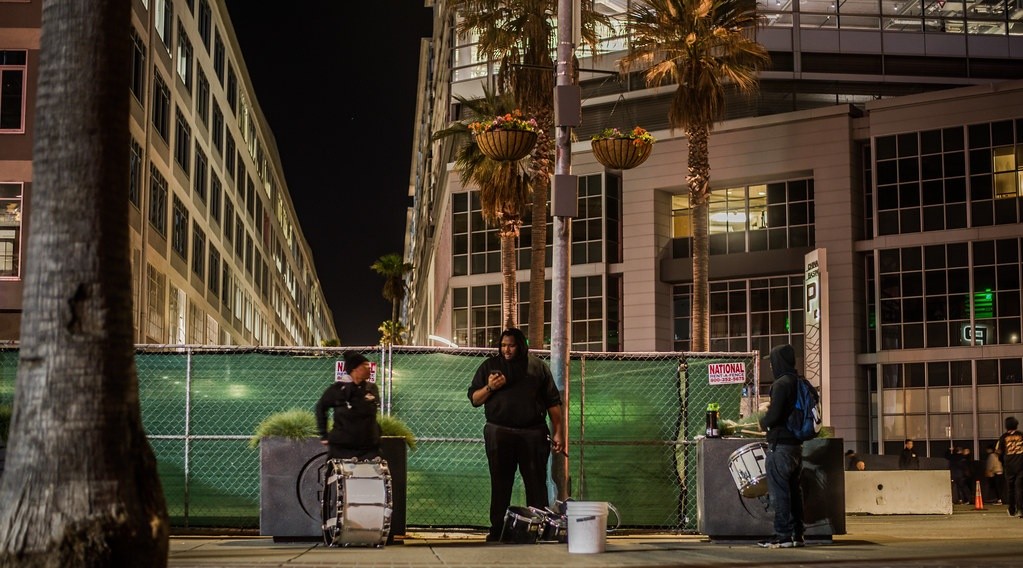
[485,531,501,542]
[1006,507,1016,517]
[993,501,1002,505]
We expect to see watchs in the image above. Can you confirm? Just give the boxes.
[485,384,493,393]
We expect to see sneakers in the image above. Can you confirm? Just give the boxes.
[792,534,806,547]
[757,533,792,548]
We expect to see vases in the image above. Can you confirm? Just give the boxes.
[474,130,538,161]
[592,138,653,170]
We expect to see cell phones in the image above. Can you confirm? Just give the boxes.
[491,370,501,376]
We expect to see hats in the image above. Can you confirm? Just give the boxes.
[1005,416,1019,430]
[343,350,369,375]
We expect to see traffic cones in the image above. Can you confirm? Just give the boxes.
[971,481,987,511]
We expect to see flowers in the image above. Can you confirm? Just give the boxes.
[467,109,543,136]
[589,126,656,156]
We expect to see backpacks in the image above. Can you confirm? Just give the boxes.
[786,375,823,439]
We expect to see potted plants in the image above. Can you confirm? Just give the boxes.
[694,412,848,536]
[248,410,417,537]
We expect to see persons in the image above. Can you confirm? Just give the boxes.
[757,344,806,548]
[845,449,865,471]
[899,439,920,470]
[946,447,1003,505]
[316,350,383,464]
[467,328,566,542]
[995,417,1023,517]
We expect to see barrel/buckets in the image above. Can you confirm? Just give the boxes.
[565,501,621,554]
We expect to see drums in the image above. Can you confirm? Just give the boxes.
[726,441,769,499]
[321,455,393,548]
[541,505,568,544]
[527,505,560,544]
[499,505,545,544]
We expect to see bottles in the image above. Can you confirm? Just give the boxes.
[705,402,720,437]
